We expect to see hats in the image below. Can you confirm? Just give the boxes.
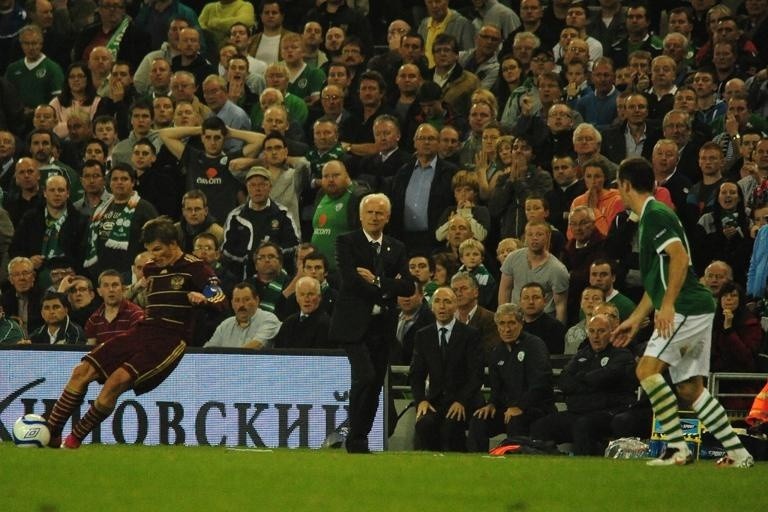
[245,165,274,181]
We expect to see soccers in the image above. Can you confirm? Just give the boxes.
[12,414,52,448]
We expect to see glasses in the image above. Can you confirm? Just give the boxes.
[479,32,501,43]
[414,135,438,142]
[500,64,518,72]
[256,253,283,263]
[68,72,87,80]
[320,94,345,101]
[568,219,593,227]
[18,37,41,49]
[193,245,212,252]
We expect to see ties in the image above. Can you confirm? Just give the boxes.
[369,241,378,272]
[439,327,447,367]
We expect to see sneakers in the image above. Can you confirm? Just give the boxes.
[645,454,696,468]
[716,456,755,468]
[58,432,82,451]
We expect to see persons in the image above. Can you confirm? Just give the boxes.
[248,62,309,127]
[555,26,579,66]
[299,21,328,68]
[10,173,90,299]
[229,133,310,239]
[79,140,108,166]
[91,116,121,177]
[602,95,661,164]
[251,89,306,140]
[435,169,490,249]
[4,157,48,227]
[335,195,415,453]
[495,220,570,325]
[135,17,212,94]
[436,127,468,172]
[185,234,238,299]
[709,281,766,456]
[743,0,767,40]
[473,124,513,211]
[389,121,458,258]
[150,96,174,129]
[641,55,680,117]
[662,110,706,170]
[271,34,326,105]
[501,47,557,127]
[605,1,662,67]
[162,116,266,225]
[541,105,578,166]
[671,87,705,135]
[533,315,633,456]
[203,284,283,349]
[313,85,355,142]
[449,276,500,351]
[419,1,474,68]
[120,254,153,310]
[54,274,99,327]
[722,79,751,101]
[4,24,65,127]
[31,105,57,132]
[72,0,150,74]
[274,253,337,318]
[222,167,299,280]
[84,162,160,287]
[512,72,564,155]
[568,160,624,240]
[751,200,768,240]
[170,28,217,104]
[91,63,143,139]
[22,293,82,348]
[27,131,82,208]
[394,276,437,366]
[108,99,163,172]
[407,288,487,453]
[706,5,731,26]
[307,160,376,287]
[164,71,214,125]
[424,35,481,112]
[336,70,409,162]
[649,141,690,214]
[489,135,556,236]
[470,306,552,455]
[513,32,541,74]
[569,121,615,186]
[545,152,586,229]
[670,7,693,37]
[712,41,745,83]
[495,136,518,177]
[0,258,51,343]
[217,56,265,131]
[687,142,731,220]
[218,21,266,75]
[748,68,767,105]
[385,65,428,124]
[703,261,733,295]
[302,116,352,200]
[316,64,357,109]
[87,47,115,101]
[239,244,296,317]
[470,0,520,41]
[710,95,766,173]
[456,25,501,89]
[355,115,413,191]
[433,217,475,275]
[407,256,439,310]
[696,17,760,64]
[694,180,751,292]
[558,205,614,282]
[41,217,227,449]
[692,67,724,128]
[558,60,600,126]
[504,0,555,52]
[127,139,180,217]
[172,101,198,129]
[199,1,255,50]
[60,110,98,168]
[456,104,498,160]
[367,20,413,89]
[278,277,334,348]
[523,195,565,258]
[610,158,752,467]
[395,32,429,74]
[1,2,65,62]
[0,133,18,193]
[399,83,466,150]
[168,191,226,254]
[331,37,368,78]
[134,0,206,52]
[141,58,175,102]
[84,270,147,348]
[555,38,592,83]
[245,2,296,63]
[519,283,566,357]
[218,43,265,94]
[493,57,540,132]
[736,139,767,216]
[564,288,611,359]
[325,27,345,57]
[662,30,696,86]
[203,75,251,153]
[553,2,603,71]
[456,239,493,300]
[303,2,370,40]
[46,62,101,139]
[579,259,650,338]
